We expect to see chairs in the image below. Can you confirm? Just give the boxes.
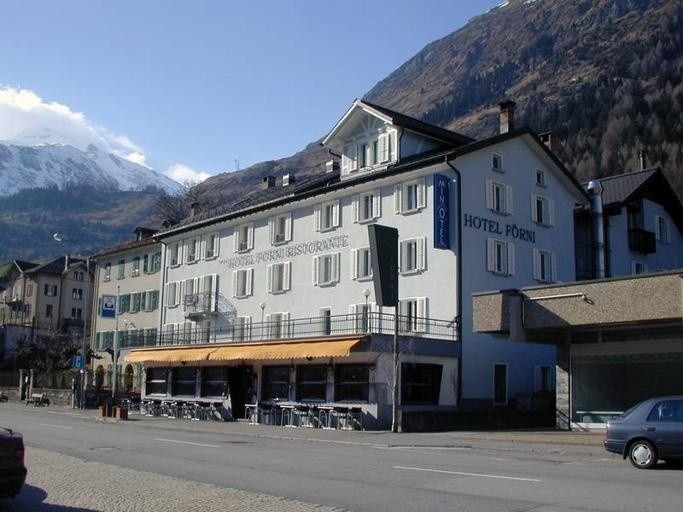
[141,394,365,430]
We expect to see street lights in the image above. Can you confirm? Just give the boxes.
[52,232,90,411]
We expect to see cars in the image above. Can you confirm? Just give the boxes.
[597,391,682,470]
[0,425,26,500]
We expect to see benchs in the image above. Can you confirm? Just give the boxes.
[25,393,50,407]
